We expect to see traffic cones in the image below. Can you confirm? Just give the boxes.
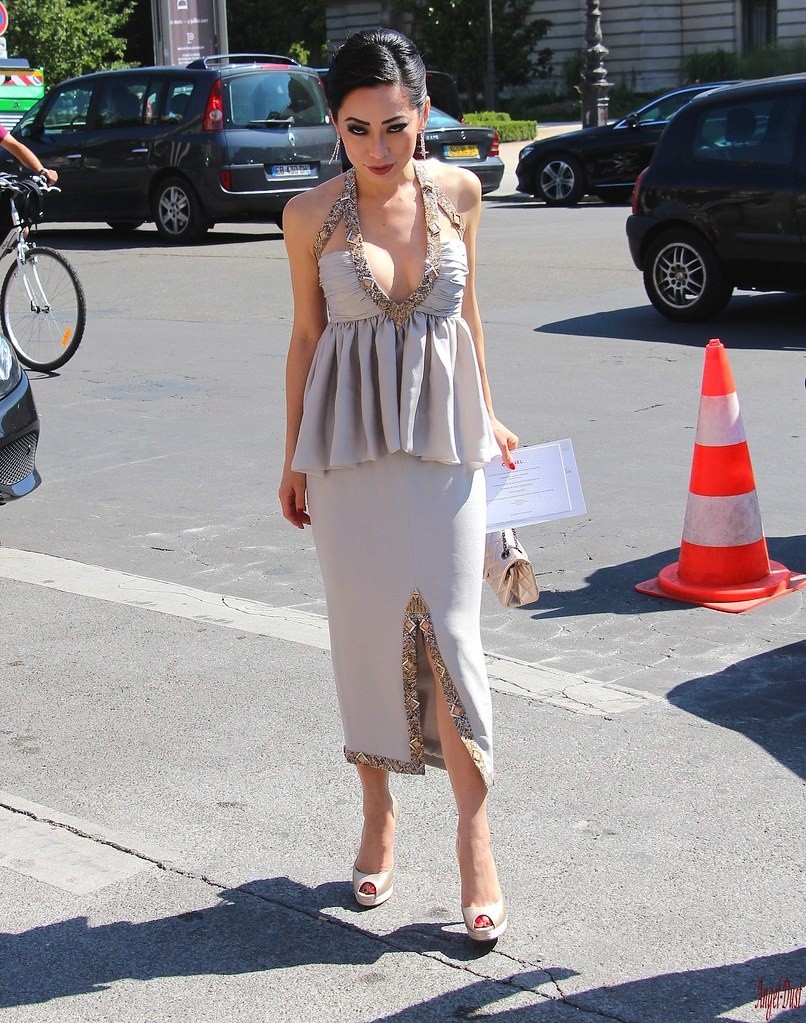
[635,337,806,616]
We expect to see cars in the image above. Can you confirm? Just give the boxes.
[515,79,746,207]
[314,68,505,195]
[0,334,42,507]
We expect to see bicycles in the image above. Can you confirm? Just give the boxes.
[0,167,87,371]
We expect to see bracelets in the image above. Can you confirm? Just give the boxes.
[39,168,47,176]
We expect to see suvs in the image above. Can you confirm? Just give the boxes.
[1,54,346,245]
[625,72,806,323]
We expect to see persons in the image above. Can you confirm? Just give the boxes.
[0,122,58,184]
[277,27,518,941]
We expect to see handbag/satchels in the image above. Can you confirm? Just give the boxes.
[482,528,540,608]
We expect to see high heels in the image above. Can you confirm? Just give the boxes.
[353,792,398,906]
[456,834,507,941]
